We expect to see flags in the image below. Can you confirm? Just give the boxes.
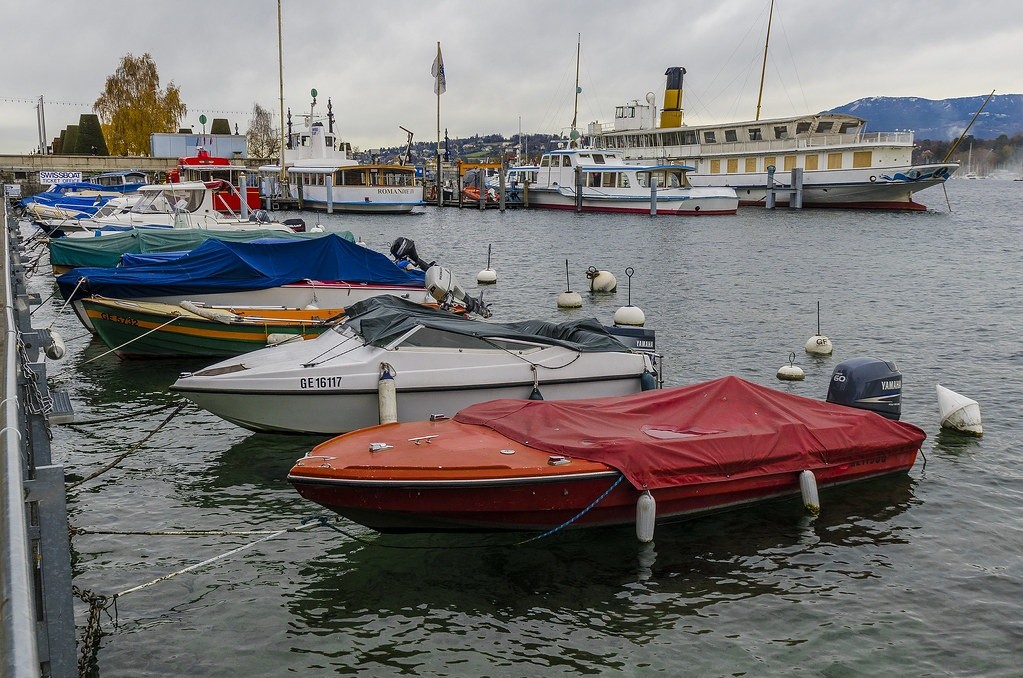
[431,51,447,94]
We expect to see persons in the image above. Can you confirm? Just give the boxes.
[488,185,496,198]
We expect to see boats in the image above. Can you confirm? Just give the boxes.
[169,292,668,434]
[58,230,446,336]
[163,149,260,211]
[18,170,324,237]
[289,358,928,533]
[484,32,741,216]
[521,0,962,212]
[82,261,493,359]
[47,228,364,280]
[260,89,426,210]
